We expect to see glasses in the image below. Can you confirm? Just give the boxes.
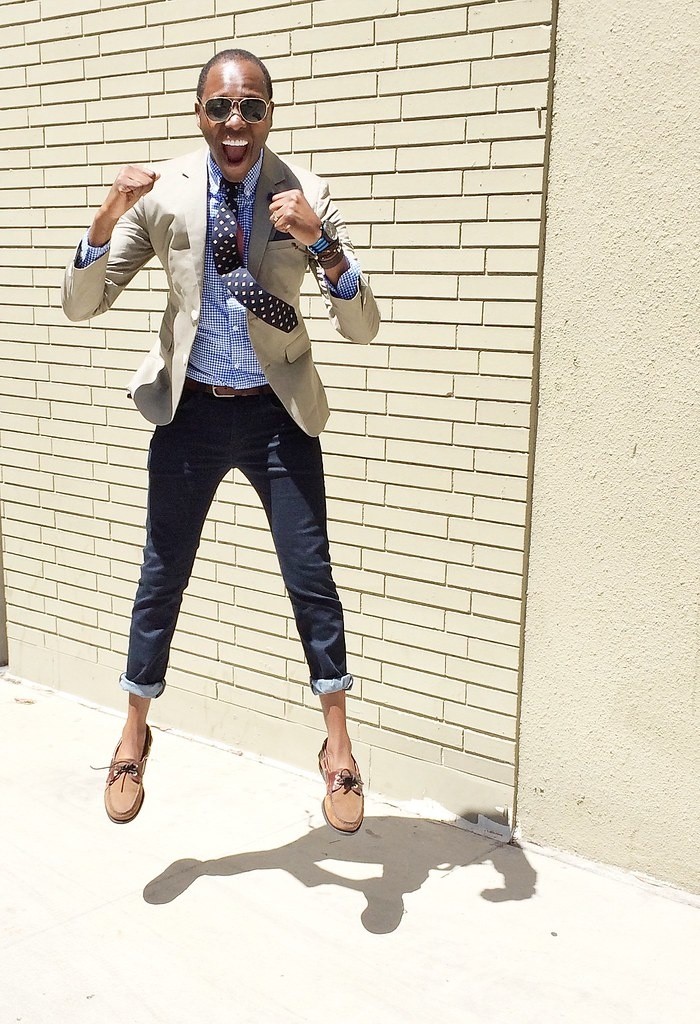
[196,95,272,124]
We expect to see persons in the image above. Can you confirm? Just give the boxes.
[60,50,380,835]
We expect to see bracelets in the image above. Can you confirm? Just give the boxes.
[318,238,343,269]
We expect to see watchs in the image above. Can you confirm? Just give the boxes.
[309,220,338,255]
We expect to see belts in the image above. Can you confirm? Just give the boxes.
[183,376,275,398]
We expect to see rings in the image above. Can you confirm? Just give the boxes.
[273,211,278,222]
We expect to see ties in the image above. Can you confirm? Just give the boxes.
[212,177,299,334]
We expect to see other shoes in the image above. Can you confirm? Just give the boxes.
[104,723,153,824]
[318,736,364,836]
